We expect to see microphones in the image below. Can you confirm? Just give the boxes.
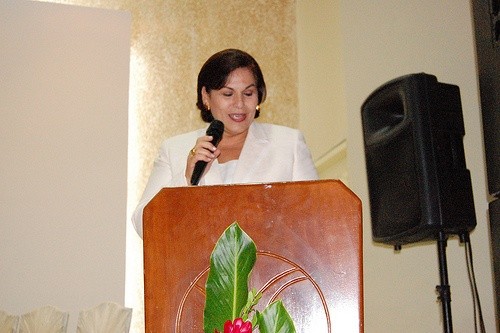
[191,120,224,185]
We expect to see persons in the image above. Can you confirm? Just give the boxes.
[132,49,319,242]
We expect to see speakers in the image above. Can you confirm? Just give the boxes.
[361,72,478,250]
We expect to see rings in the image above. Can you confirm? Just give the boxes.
[191,147,196,156]
